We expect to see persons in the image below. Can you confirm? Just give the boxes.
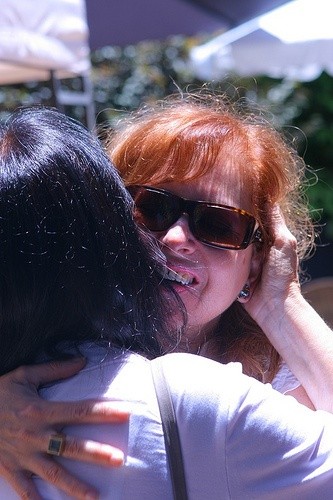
[0,103,333,500]
[0,80,324,500]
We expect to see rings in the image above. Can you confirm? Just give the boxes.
[47,431,67,456]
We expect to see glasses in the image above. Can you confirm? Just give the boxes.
[125,184,266,251]
